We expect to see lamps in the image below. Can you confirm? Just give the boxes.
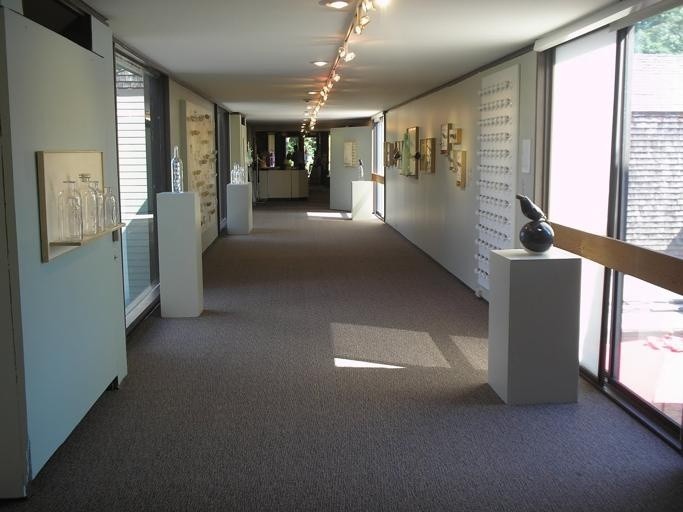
[308,1,376,130]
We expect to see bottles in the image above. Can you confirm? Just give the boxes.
[231,164,244,185]
[59,172,117,243]
[171,145,183,193]
